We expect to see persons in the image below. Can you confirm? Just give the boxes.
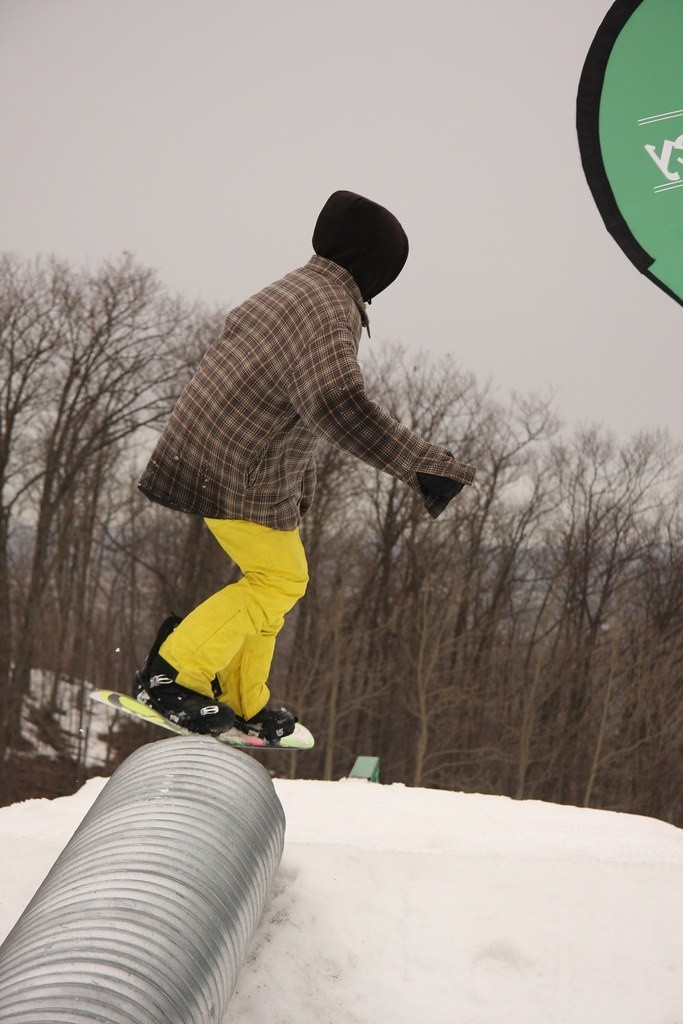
[133,189,476,745]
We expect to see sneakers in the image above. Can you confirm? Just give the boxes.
[224,700,316,750]
[133,667,236,736]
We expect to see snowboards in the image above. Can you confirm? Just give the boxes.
[87,688,315,751]
